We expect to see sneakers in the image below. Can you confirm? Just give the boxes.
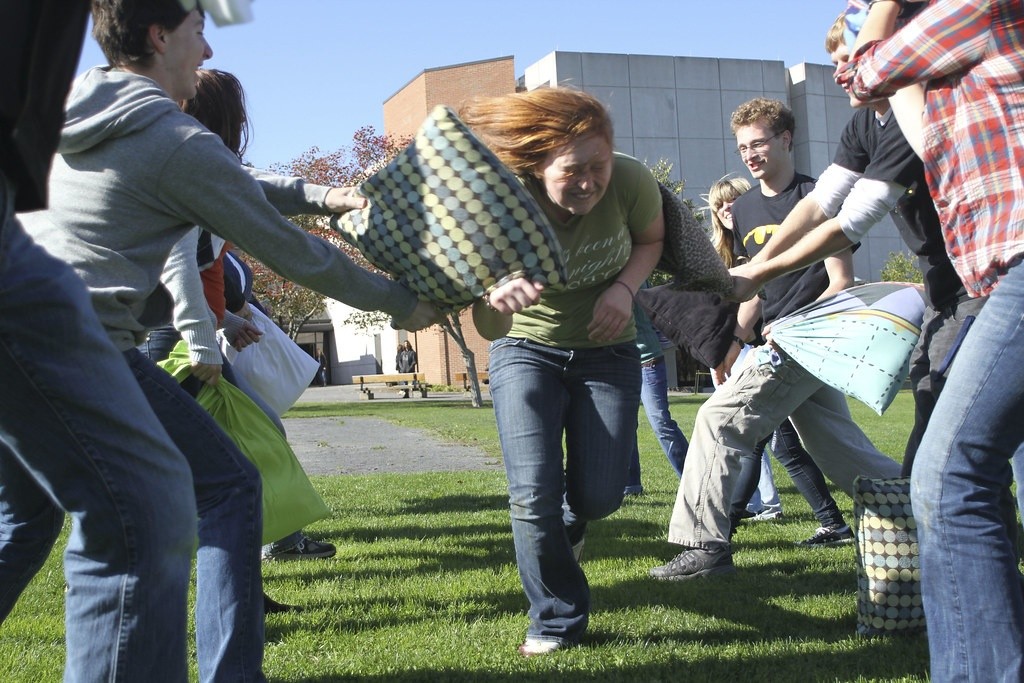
[646,548,734,584]
[269,538,336,562]
[799,526,855,548]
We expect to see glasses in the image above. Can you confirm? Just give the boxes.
[734,131,783,155]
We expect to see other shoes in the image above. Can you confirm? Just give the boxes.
[267,601,303,614]
[573,539,584,563]
[517,639,561,658]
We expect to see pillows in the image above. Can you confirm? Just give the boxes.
[637,279,739,370]
[212,298,323,418]
[654,176,737,298]
[771,280,932,416]
[329,101,570,331]
[156,338,333,546]
[854,474,928,637]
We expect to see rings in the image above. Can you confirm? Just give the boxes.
[254,336,259,342]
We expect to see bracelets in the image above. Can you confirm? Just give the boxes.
[485,294,497,312]
[732,335,744,349]
[614,280,636,303]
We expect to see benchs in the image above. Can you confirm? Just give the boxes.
[352,372,427,400]
[455,372,492,396]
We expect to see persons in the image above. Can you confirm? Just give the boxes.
[216,250,335,560]
[396,340,417,398]
[649,99,902,582]
[625,175,857,546]
[138,69,304,617]
[833,0,1024,682]
[727,12,1020,566]
[317,348,329,387]
[0,0,203,683]
[15,0,439,683]
[462,88,663,654]
